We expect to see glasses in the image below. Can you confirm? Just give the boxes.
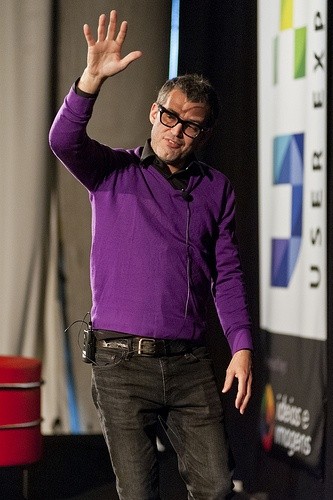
[158,104,208,139]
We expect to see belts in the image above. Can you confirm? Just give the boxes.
[99,337,189,358]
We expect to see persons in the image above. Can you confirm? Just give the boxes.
[48,9,253,500]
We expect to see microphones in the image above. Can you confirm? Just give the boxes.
[182,192,193,202]
